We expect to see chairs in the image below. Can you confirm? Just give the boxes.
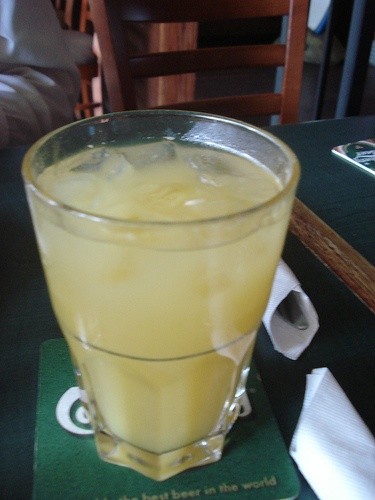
[89,0,309,125]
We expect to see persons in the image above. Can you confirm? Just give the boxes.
[0,0,80,163]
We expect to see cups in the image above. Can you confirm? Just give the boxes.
[19,109,301,481]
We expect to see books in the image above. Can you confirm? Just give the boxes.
[331,139,375,176]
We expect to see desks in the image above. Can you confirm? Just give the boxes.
[1,116,375,499]
